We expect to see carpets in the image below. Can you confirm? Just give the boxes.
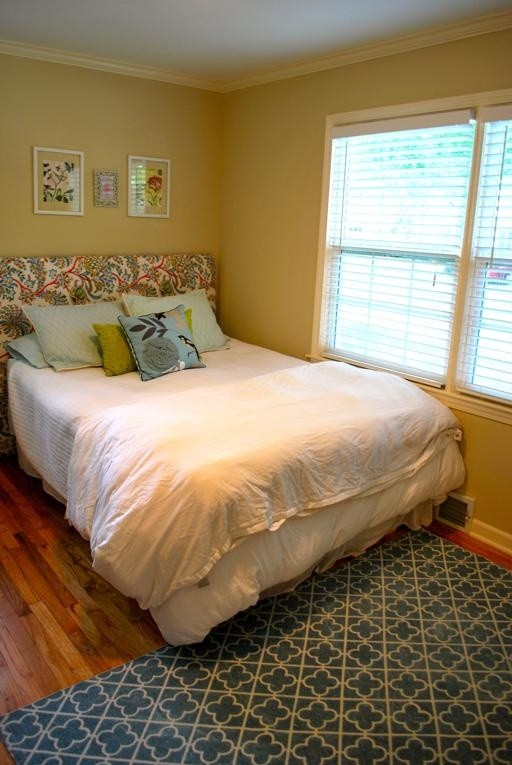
[1,521,511,765]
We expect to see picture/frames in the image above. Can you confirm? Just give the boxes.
[93,167,122,208]
[127,154,170,219]
[32,145,85,216]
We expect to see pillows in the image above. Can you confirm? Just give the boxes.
[5,289,231,383]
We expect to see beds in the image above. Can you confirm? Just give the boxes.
[0,253,468,641]
